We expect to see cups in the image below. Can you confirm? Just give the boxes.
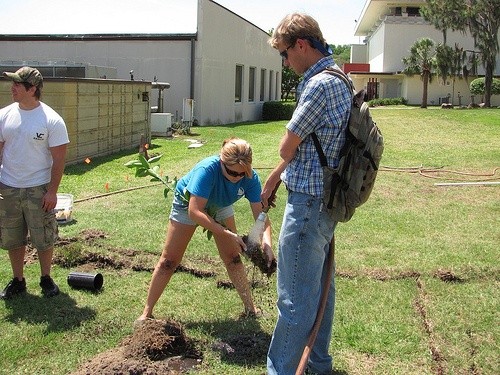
[67,272,103,290]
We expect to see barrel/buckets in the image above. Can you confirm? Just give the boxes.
[54,193,74,224]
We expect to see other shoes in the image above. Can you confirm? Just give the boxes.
[39,274,60,298]
[0,276,27,300]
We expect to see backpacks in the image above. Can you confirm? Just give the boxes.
[293,66,385,224]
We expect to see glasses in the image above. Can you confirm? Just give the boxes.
[280,44,292,60]
[223,163,246,177]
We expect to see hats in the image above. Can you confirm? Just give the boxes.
[2,67,44,90]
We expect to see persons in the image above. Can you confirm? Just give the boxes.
[134,136,275,327]
[260,12,355,375]
[0,65,71,302]
